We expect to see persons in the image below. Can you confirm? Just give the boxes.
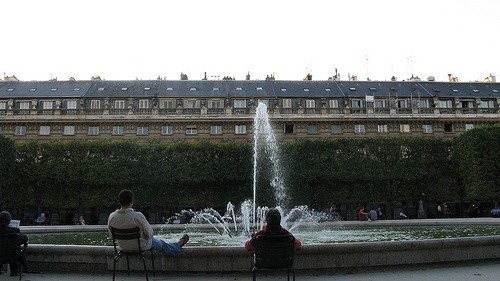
[162,209,199,224]
[245,209,301,269]
[330,205,339,214]
[377,208,386,219]
[0,211,29,281]
[76,216,86,225]
[37,213,47,222]
[490,206,500,217]
[400,210,407,219]
[108,190,189,256]
[359,207,368,220]
[369,208,377,221]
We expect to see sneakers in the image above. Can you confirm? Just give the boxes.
[10,272,18,277]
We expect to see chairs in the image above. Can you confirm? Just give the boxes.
[247,233,296,281]
[110,226,156,281]
[0,234,28,280]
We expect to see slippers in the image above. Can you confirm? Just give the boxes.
[178,234,189,248]
